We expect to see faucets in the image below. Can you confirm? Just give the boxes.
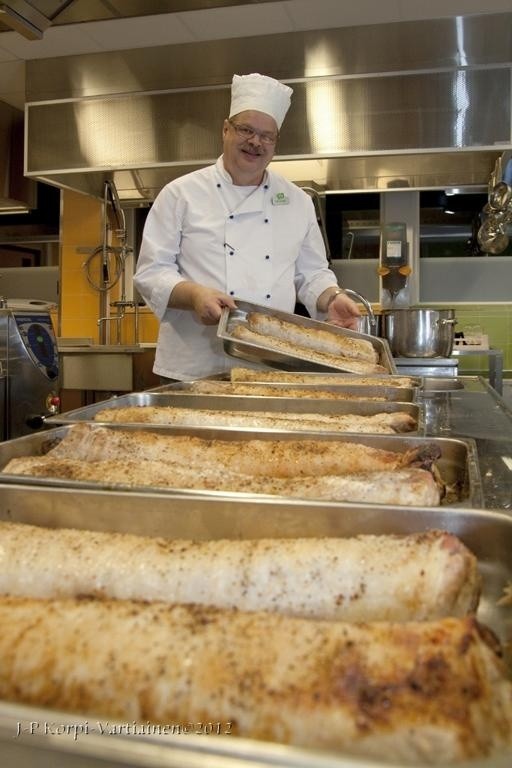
[97,311,124,325]
[324,287,376,333]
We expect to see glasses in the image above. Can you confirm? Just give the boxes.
[228,120,276,146]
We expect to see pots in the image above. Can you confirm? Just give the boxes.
[381,308,459,357]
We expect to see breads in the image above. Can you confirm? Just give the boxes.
[94,407,399,435]
[229,325,390,377]
[158,407,420,431]
[1,517,486,623]
[3,454,444,508]
[230,367,418,389]
[44,422,408,479]
[0,592,510,766]
[246,312,380,364]
[190,378,390,403]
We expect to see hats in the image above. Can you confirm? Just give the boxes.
[229,73,294,137]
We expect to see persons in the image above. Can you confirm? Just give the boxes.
[134,108,362,389]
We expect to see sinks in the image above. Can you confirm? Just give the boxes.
[58,342,154,392]
